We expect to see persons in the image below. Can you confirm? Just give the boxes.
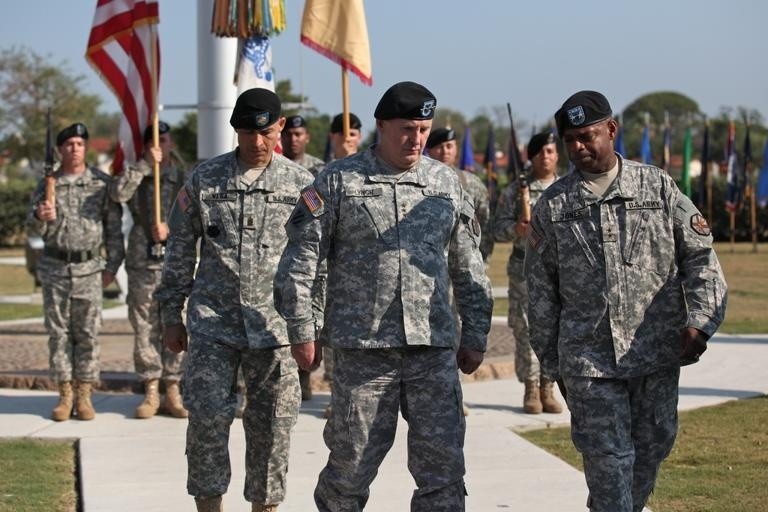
[153,84,326,511]
[494,129,565,413]
[271,79,493,511]
[280,114,324,402]
[24,123,126,420]
[523,89,729,510]
[429,125,491,418]
[108,122,190,419]
[326,107,361,164]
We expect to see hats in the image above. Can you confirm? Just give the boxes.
[57,123,90,145]
[425,128,456,149]
[555,91,613,130]
[331,113,361,134]
[528,131,556,160]
[229,88,281,131]
[284,115,307,130]
[372,81,437,121]
[143,120,169,143]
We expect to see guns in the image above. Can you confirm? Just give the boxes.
[507,103,531,222]
[43,107,55,206]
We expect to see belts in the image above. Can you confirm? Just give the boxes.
[512,244,526,260]
[45,244,97,265]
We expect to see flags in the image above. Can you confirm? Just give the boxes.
[233,34,277,99]
[299,1,374,90]
[84,0,162,174]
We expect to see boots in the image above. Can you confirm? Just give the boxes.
[235,385,251,420]
[163,378,190,418]
[135,378,161,418]
[541,380,563,414]
[295,363,314,401]
[523,381,543,415]
[52,381,75,421]
[74,382,95,420]
